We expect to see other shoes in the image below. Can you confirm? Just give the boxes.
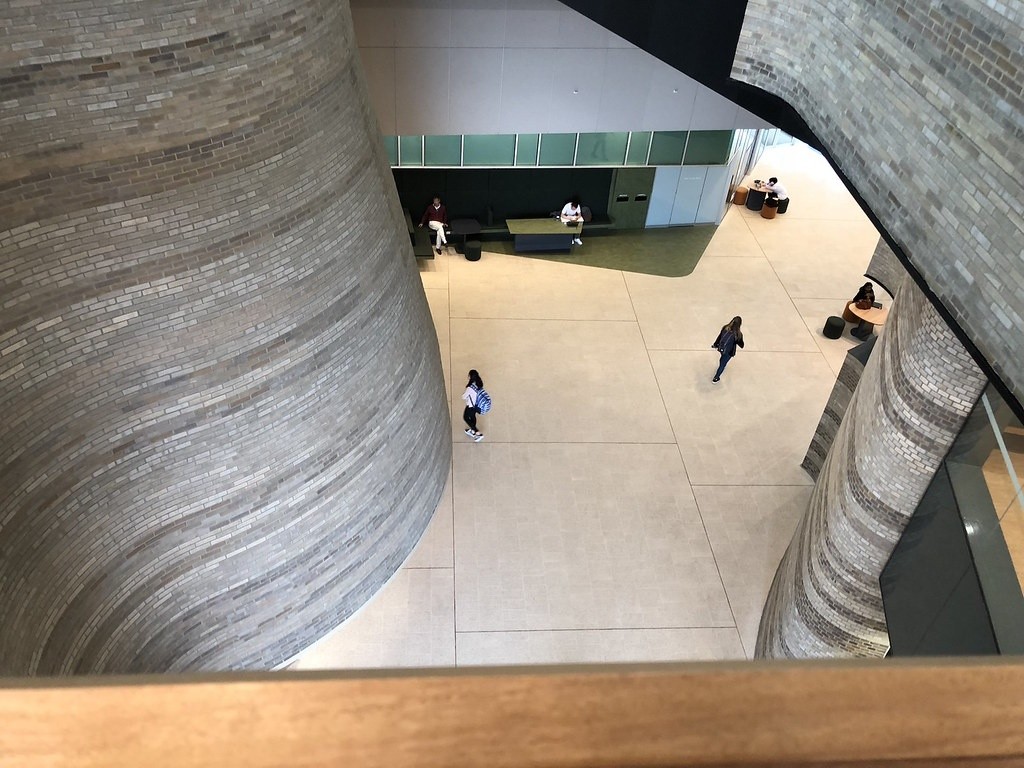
[574,237,583,246]
[572,240,574,245]
[711,377,720,384]
[445,244,448,248]
[436,248,442,255]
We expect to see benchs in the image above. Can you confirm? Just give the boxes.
[414,214,614,235]
[414,225,434,259]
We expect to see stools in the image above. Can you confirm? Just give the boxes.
[465,240,481,261]
[823,316,845,339]
[872,302,882,310]
[734,187,748,205]
[777,198,789,213]
[761,201,779,219]
[843,301,859,323]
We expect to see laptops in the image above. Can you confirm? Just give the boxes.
[566,222,580,227]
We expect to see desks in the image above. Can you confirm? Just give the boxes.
[849,302,888,341]
[746,182,773,211]
[506,218,581,252]
[450,218,481,254]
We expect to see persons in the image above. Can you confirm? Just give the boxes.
[418,195,448,255]
[561,200,583,245]
[712,315,744,384]
[462,369,485,442]
[853,282,875,305]
[766,176,788,200]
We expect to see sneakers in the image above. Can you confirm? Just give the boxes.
[474,433,484,442]
[464,428,476,438]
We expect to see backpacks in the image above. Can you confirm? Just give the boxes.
[716,328,738,356]
[466,383,492,415]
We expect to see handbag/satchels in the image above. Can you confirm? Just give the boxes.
[855,296,871,310]
[766,195,779,206]
